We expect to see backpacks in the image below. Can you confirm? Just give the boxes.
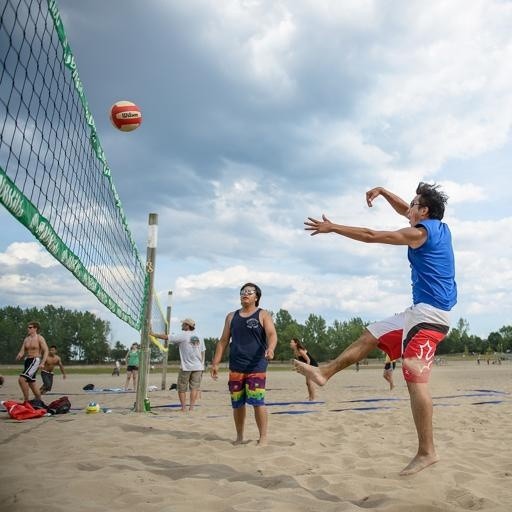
[49,396,71,413]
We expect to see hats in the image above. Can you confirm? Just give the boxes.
[181,319,196,328]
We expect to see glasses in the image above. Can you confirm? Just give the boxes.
[410,201,424,208]
[240,289,256,295]
[28,327,32,329]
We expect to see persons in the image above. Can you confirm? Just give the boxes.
[290,338,319,401]
[290,181,458,475]
[123,343,139,392]
[382,351,397,391]
[150,318,206,412]
[111,358,121,376]
[16,321,49,402]
[38,346,66,394]
[210,283,278,447]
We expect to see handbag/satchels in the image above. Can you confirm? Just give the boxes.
[2,400,46,420]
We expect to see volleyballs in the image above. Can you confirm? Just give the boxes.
[108,100,142,132]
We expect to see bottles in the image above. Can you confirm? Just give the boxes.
[145,397,151,412]
